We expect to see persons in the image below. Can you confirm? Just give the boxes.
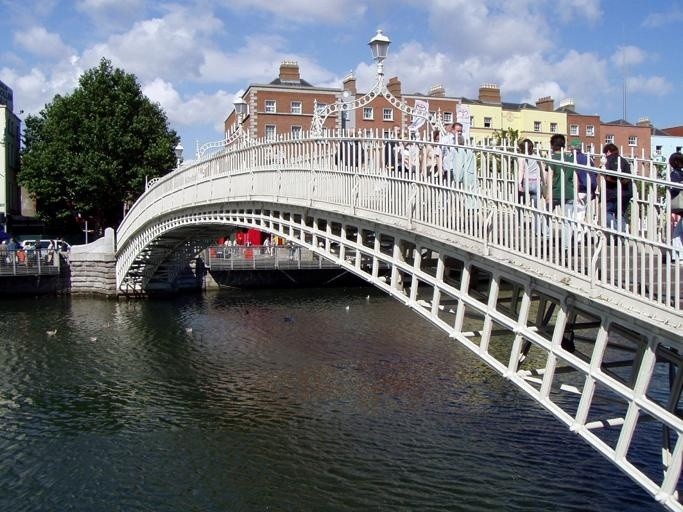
[665,153,683,267]
[60,238,68,251]
[263,236,271,254]
[224,238,232,246]
[514,134,632,256]
[335,132,365,167]
[232,239,237,246]
[383,123,476,209]
[0,238,42,267]
[44,240,54,265]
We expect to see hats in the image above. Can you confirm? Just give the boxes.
[571,139,581,149]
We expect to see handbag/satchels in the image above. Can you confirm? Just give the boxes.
[606,175,631,184]
[666,182,683,213]
[522,179,543,194]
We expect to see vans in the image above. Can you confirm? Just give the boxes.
[21,239,71,255]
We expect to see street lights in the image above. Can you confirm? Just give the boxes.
[78,211,88,244]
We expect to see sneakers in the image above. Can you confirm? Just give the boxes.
[672,259,682,266]
